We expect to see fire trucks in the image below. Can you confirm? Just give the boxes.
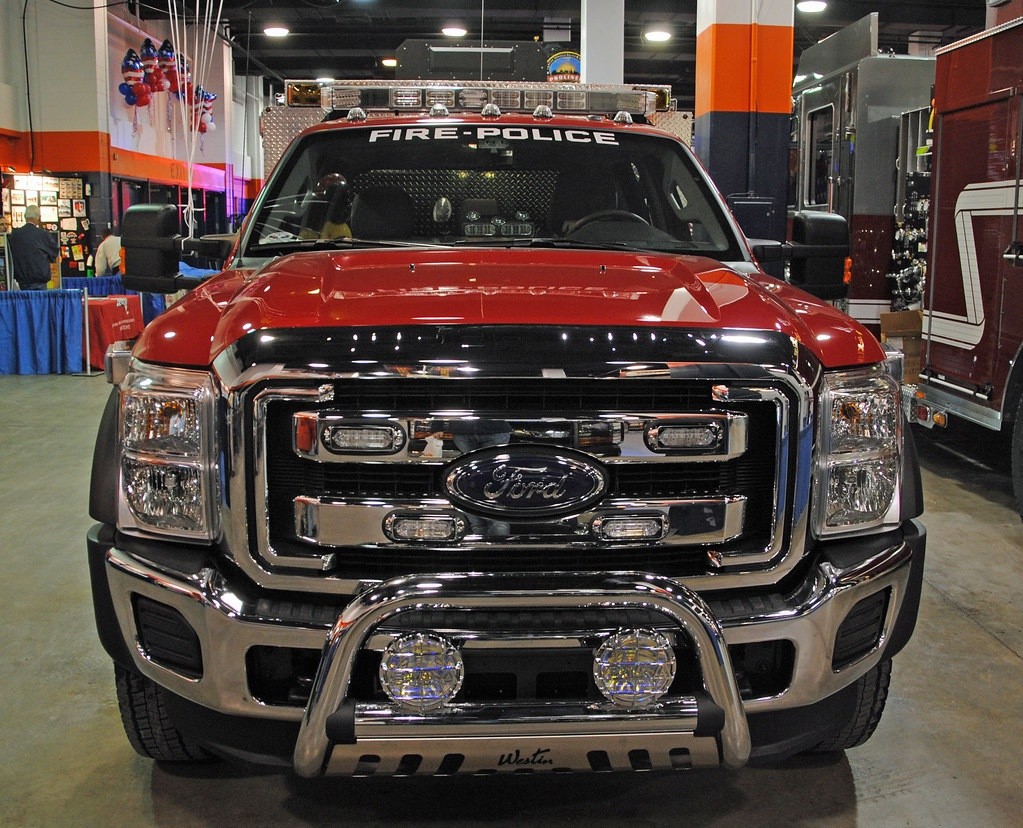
[786,0,1023,519]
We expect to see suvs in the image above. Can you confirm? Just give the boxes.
[87,106,927,782]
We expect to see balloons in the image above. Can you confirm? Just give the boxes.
[119,36,217,134]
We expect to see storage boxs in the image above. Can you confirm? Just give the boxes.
[879,308,922,385]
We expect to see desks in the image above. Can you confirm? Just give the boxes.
[71,294,145,373]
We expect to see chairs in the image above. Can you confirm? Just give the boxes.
[547,166,630,241]
[348,186,416,244]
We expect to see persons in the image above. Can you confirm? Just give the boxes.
[6,204,59,290]
[95,227,122,276]
[0,216,9,233]
